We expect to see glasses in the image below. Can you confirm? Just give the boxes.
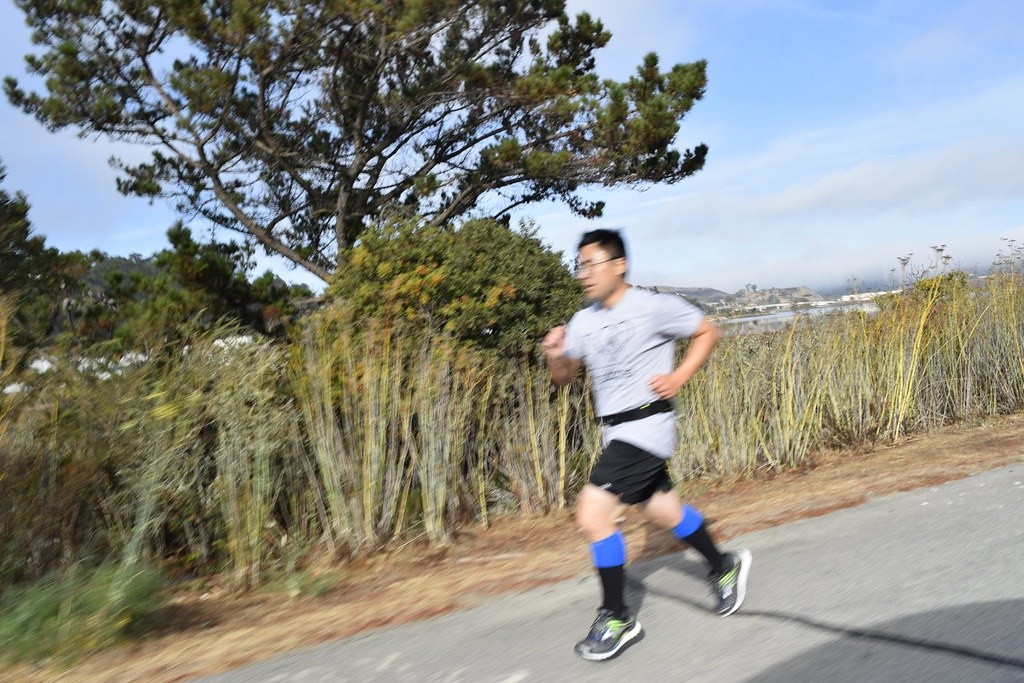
[580,255,617,268]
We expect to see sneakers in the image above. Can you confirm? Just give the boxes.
[575,606,642,661]
[706,547,752,618]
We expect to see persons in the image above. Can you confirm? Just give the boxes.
[541,228,753,661]
